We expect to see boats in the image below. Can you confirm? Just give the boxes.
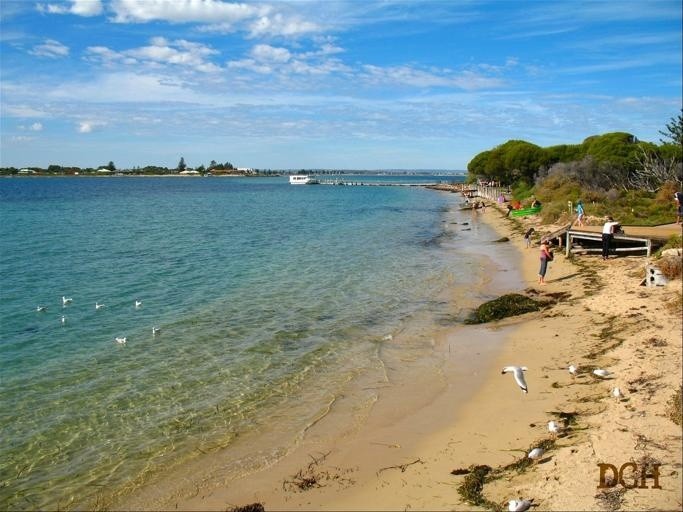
[458,201,479,209]
[505,204,542,219]
[287,174,319,186]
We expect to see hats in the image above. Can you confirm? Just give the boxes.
[541,237,547,244]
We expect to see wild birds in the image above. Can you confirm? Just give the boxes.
[612,387,623,403]
[506,497,539,511]
[547,420,564,440]
[501,365,531,395]
[591,368,616,380]
[134,299,143,306]
[152,326,161,333]
[568,364,583,378]
[95,302,104,309]
[114,336,129,344]
[36,305,47,311]
[62,295,72,304]
[529,446,548,463]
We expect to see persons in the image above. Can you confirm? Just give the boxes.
[465,180,543,219]
[675,192,683,229]
[602,217,624,262]
[537,236,554,287]
[574,200,586,227]
[524,228,535,250]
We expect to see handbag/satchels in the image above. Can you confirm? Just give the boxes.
[546,252,553,261]
